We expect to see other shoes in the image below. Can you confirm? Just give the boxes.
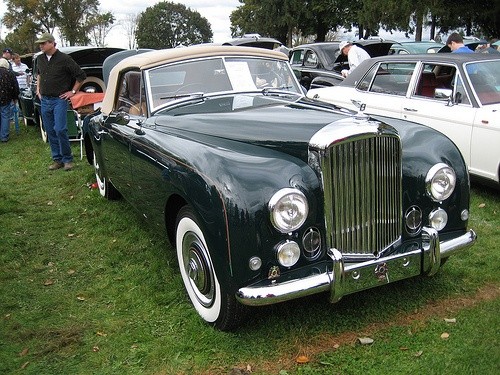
[63,162,73,171]
[50,160,64,170]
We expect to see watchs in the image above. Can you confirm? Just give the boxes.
[71,89,77,94]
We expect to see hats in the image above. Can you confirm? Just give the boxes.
[35,33,55,43]
[2,48,15,55]
[339,40,349,53]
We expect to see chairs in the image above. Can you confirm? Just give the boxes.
[129,102,146,116]
[416,73,438,97]
[66,100,84,160]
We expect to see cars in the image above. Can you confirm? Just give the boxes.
[305,53,500,188]
[82,44,478,334]
[17,32,500,143]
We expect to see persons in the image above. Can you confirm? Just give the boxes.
[11,52,35,125]
[0,57,19,142]
[32,32,89,170]
[0,48,24,119]
[338,41,372,77]
[447,33,480,87]
